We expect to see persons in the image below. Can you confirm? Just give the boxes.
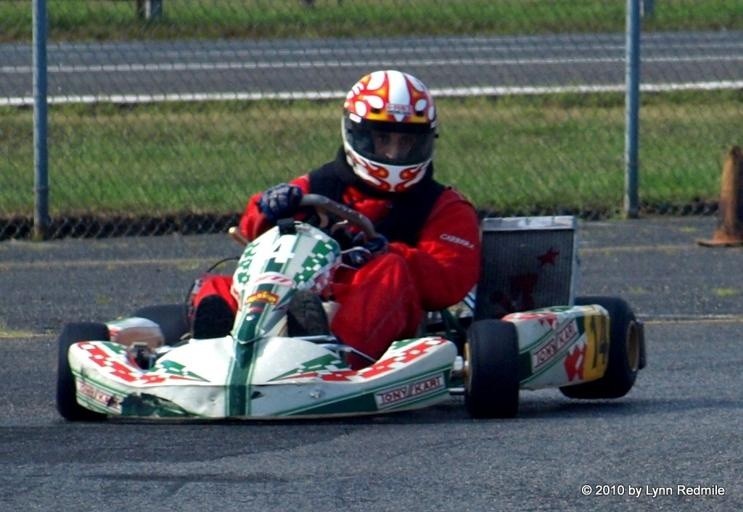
[186,70,484,370]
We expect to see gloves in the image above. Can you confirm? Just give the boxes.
[255,181,305,219]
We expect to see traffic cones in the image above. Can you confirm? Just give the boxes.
[692,144,743,248]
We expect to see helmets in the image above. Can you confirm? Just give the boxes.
[341,71,439,194]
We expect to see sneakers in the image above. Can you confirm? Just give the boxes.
[286,289,330,337]
[190,291,234,338]
